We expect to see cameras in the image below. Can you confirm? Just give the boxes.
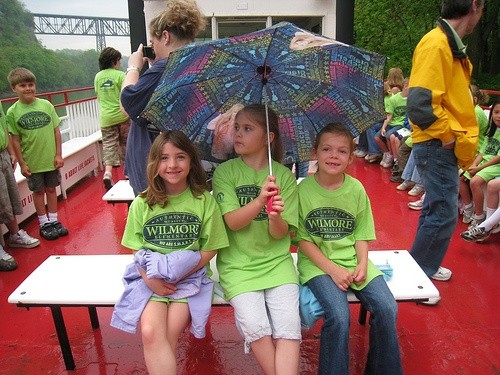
[141,46,155,59]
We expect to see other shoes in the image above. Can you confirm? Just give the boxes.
[420,298,442,306]
[8,228,41,248]
[475,223,500,243]
[50,221,69,236]
[0,245,14,261]
[408,191,428,210]
[468,217,486,231]
[407,183,425,197]
[39,222,60,240]
[433,265,453,282]
[395,182,412,192]
[388,161,403,176]
[390,175,403,184]
[364,152,384,163]
[102,173,114,190]
[379,152,393,168]
[461,202,474,224]
[459,225,492,241]
[353,149,366,158]
[0,258,19,272]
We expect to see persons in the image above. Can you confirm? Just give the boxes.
[211,104,304,375]
[296,122,405,375]
[353,65,500,243]
[0,100,41,262]
[6,68,69,241]
[119,0,207,199]
[121,129,230,375]
[402,0,485,305]
[94,47,127,189]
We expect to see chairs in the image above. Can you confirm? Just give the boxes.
[101,178,137,211]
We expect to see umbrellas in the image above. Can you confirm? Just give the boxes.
[137,21,389,213]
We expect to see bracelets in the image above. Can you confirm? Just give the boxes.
[126,65,141,73]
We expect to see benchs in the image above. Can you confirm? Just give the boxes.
[60,135,99,200]
[87,131,106,169]
[7,248,441,373]
[0,162,61,248]
